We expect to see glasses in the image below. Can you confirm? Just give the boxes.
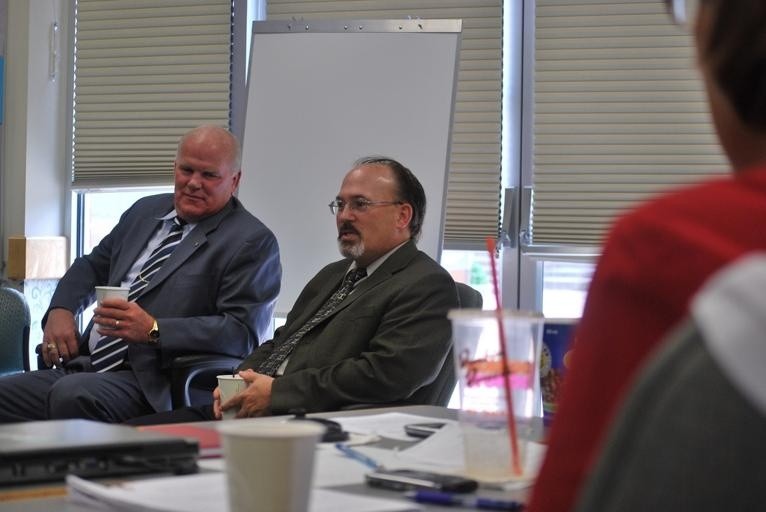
[329,199,403,215]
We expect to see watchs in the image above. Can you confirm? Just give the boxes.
[148,319,160,345]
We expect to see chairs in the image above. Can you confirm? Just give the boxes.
[32,340,248,410]
[412,280,483,409]
[0,282,35,377]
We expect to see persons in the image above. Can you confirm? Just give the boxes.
[0,123,282,421]
[521,0,765,511]
[117,155,461,427]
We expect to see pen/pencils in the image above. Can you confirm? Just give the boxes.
[335,442,386,470]
[403,489,525,509]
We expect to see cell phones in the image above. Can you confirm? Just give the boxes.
[366,470,478,493]
[403,420,446,438]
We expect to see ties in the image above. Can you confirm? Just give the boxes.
[253,267,367,377]
[90,216,187,374]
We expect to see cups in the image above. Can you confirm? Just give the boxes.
[216,420,327,512]
[446,308,546,484]
[94,285,132,331]
[216,373,253,420]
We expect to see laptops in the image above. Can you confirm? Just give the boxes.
[0,417,201,484]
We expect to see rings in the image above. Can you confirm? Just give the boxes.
[115,319,119,330]
[46,342,55,352]
